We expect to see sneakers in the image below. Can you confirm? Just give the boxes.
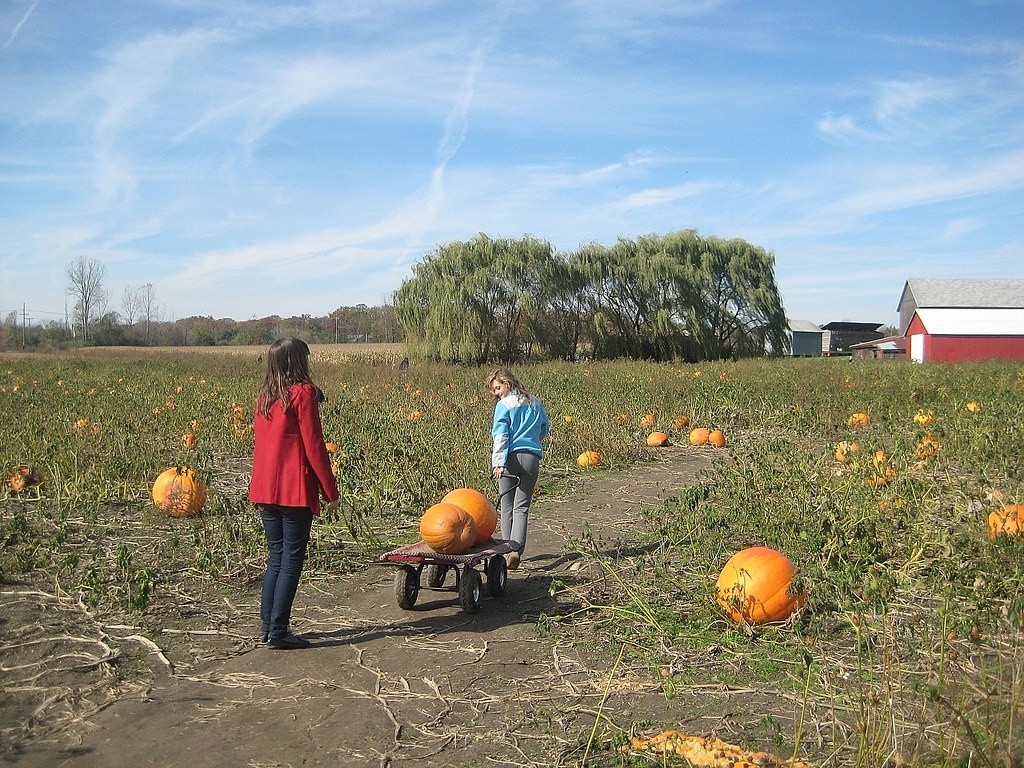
[506,551,520,569]
[261,630,310,649]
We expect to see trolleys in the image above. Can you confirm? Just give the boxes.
[381,472,528,616]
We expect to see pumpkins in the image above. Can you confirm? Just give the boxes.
[153,465,208,519]
[574,412,726,467]
[989,504,1024,542]
[835,400,983,485]
[714,547,808,627]
[441,489,497,547]
[419,503,478,554]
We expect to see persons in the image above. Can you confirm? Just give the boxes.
[240,335,339,652]
[481,367,551,569]
